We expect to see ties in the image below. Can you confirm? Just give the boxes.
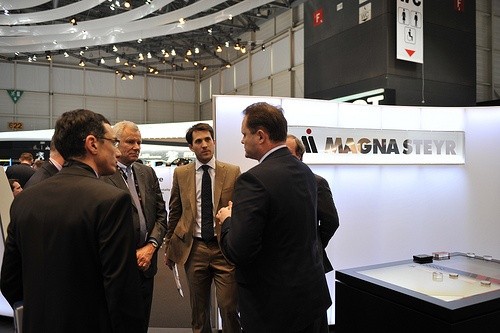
[126,166,146,247]
[201,165,214,244]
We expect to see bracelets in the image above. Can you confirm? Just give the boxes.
[148,241,157,248]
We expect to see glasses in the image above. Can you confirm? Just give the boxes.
[81,136,120,148]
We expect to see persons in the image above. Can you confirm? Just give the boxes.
[23,134,65,189]
[0,109,141,333]
[98,120,168,333]
[33,160,45,170]
[216,103,332,333]
[5,152,36,189]
[283,136,339,274]
[8,179,24,197]
[164,123,241,333]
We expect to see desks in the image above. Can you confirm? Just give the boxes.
[333,252,500,332]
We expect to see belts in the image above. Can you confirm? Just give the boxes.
[194,236,217,242]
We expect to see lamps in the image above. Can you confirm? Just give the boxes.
[70,17,76,25]
[14,28,266,81]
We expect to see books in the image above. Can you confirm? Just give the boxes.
[13,305,23,333]
